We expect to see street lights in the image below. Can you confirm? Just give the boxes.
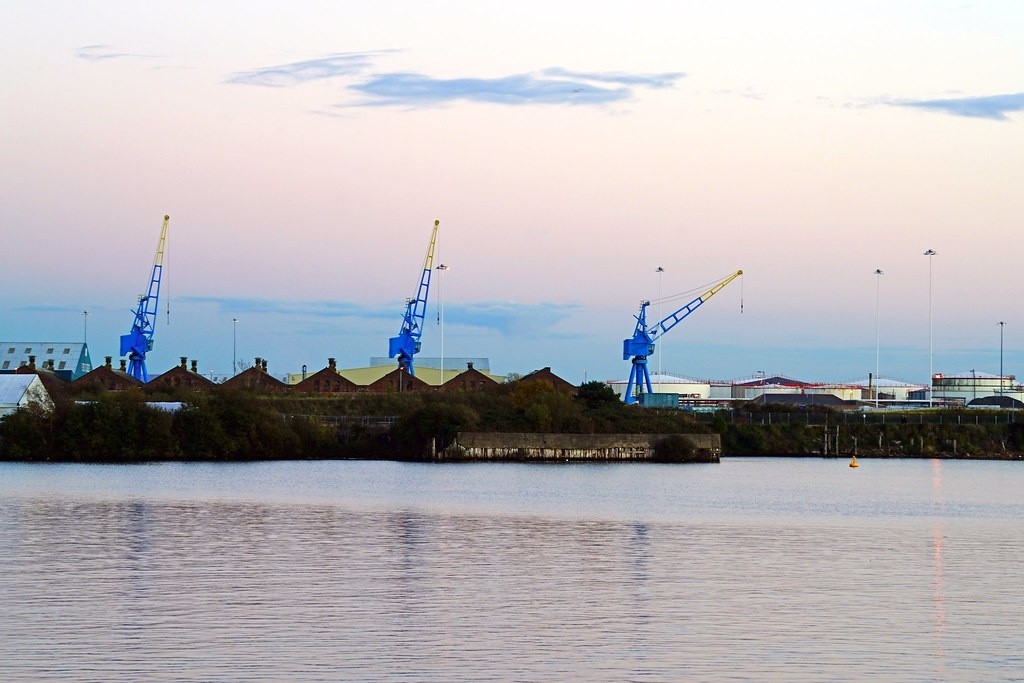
[969,368,976,399]
[302,364,307,380]
[81,310,89,342]
[923,248,938,409]
[873,269,886,409]
[231,318,239,376]
[995,321,1007,397]
[756,370,766,403]
[211,369,213,384]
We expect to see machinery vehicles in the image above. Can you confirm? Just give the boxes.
[120,214,171,384]
[619,269,745,396]
[390,217,449,379]
